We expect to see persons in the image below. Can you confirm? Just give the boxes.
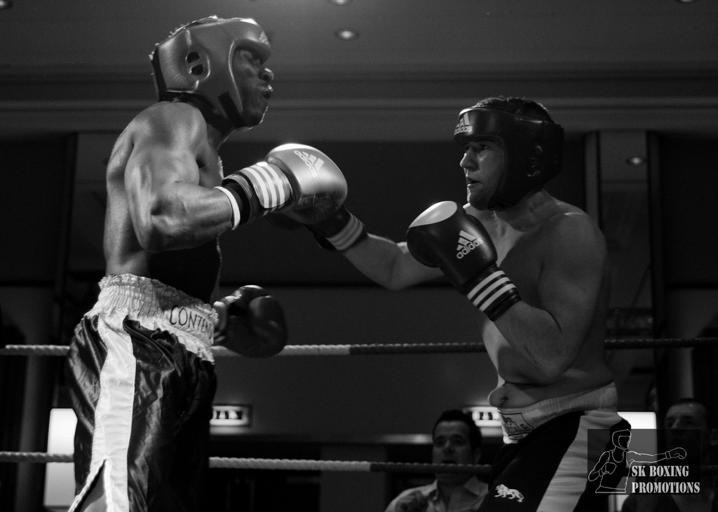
[301,95,631,512]
[382,407,489,512]
[59,14,348,512]
[619,397,717,511]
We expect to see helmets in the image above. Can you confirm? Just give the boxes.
[452,106,569,213]
[150,17,271,130]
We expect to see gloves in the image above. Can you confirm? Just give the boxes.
[406,200,521,322]
[212,283,288,358]
[215,141,364,253]
